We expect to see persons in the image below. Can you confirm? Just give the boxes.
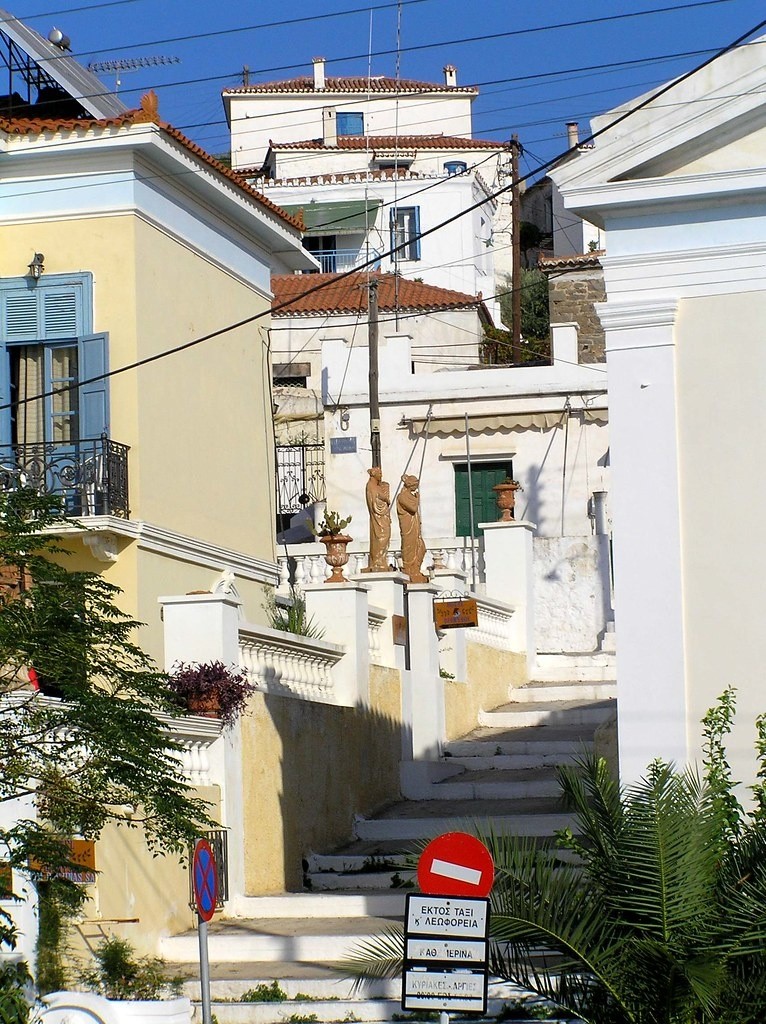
[396,474,429,583]
[366,467,393,568]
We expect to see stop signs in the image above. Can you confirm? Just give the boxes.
[418,830,495,898]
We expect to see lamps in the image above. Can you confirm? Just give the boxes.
[28,253,44,278]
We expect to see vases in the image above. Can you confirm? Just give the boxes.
[188,698,220,718]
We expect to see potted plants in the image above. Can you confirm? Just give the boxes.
[492,478,524,522]
[316,508,354,581]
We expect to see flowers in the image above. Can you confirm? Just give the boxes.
[164,662,257,728]
